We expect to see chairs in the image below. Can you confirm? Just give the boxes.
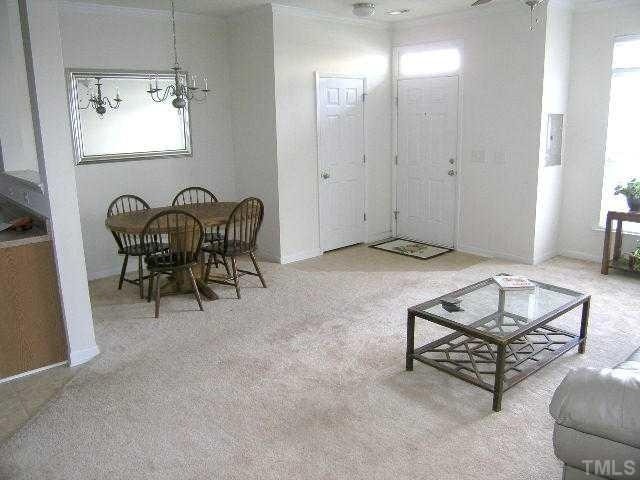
[106,187,267,318]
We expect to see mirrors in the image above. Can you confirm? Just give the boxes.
[64,69,194,166]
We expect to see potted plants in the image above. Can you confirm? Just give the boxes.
[613,176,640,211]
[623,240,640,272]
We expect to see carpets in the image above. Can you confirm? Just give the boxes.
[367,236,456,261]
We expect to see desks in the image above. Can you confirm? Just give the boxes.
[600,207,640,275]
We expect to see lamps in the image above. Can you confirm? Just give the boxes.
[146,1,212,116]
[76,76,124,120]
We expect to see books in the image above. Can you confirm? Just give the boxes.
[491,275,536,292]
